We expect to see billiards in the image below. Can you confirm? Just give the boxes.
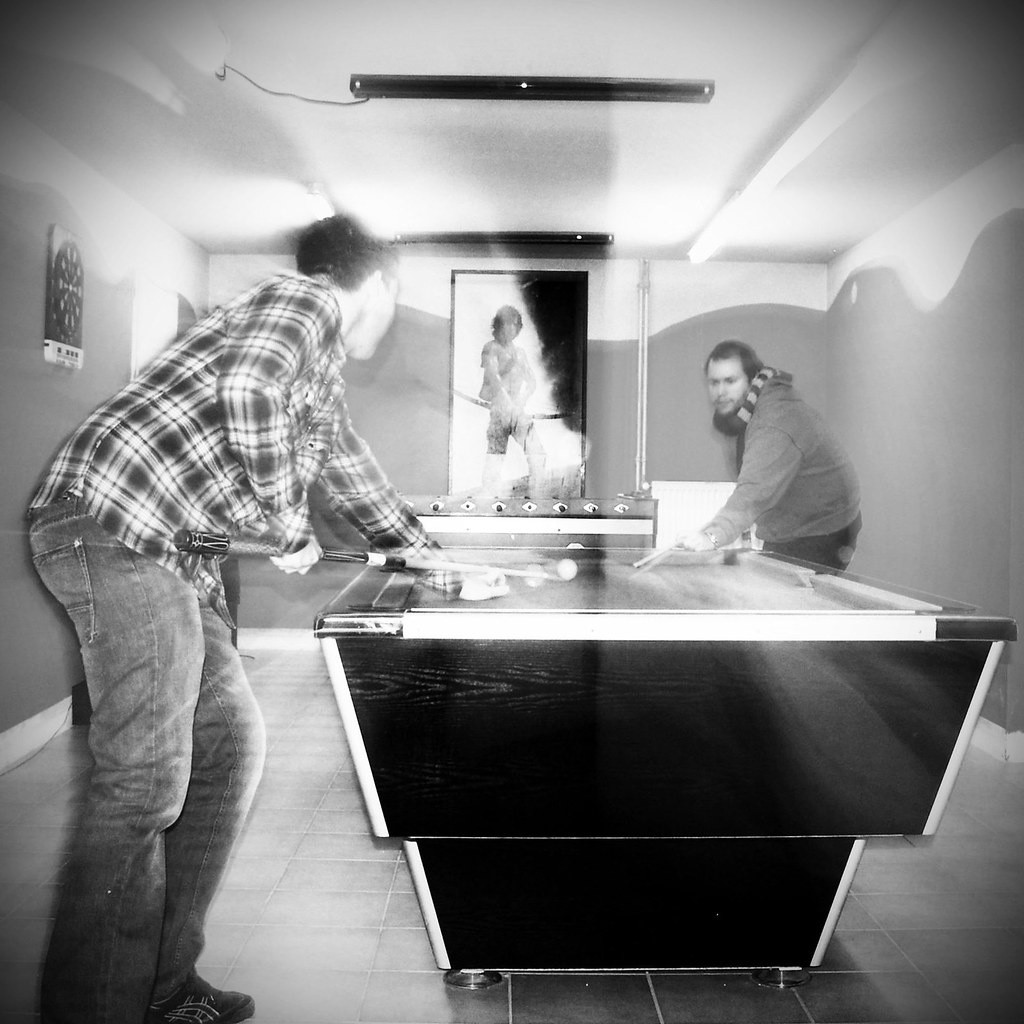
[555,557,580,581]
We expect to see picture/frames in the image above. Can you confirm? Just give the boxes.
[447,270,589,500]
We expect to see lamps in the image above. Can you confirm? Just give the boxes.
[349,73,716,107]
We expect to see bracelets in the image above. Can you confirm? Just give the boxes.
[706,533,720,550]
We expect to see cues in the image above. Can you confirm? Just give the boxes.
[635,257,652,492]
[631,534,688,569]
[171,526,559,585]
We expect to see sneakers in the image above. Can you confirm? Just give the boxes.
[145,972,254,1024]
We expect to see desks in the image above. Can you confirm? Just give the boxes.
[311,542,1018,989]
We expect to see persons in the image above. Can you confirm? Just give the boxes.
[675,340,862,570]
[22,214,509,1024]
[482,305,547,486]
